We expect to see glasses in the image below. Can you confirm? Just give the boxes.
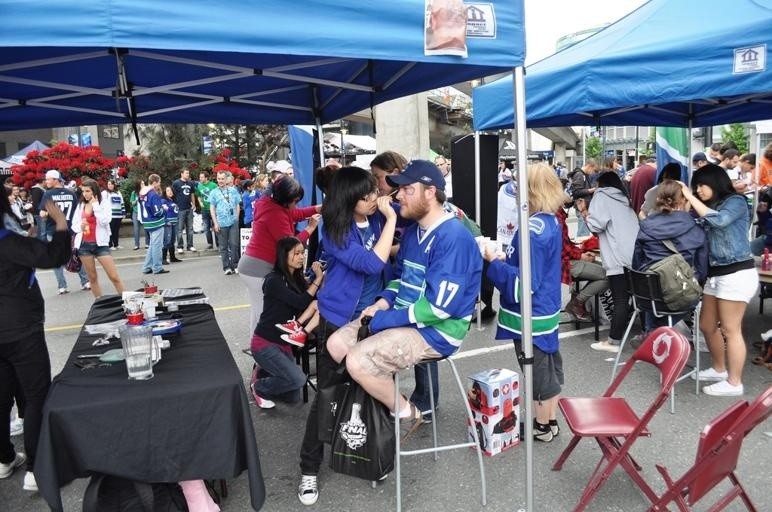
[359,186,379,202]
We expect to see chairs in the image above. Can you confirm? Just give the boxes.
[609,266,701,414]
[371,294,486,511]
[646,385,772,512]
[548,325,691,512]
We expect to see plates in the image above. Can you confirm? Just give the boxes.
[145,319,184,333]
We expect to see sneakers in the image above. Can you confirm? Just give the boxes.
[548,421,561,435]
[279,327,308,349]
[249,380,277,411]
[22,469,41,491]
[187,246,198,252]
[223,266,240,276]
[589,340,621,353]
[517,422,551,444]
[700,377,744,397]
[177,246,185,254]
[384,402,422,447]
[418,411,438,425]
[274,317,304,335]
[9,415,27,437]
[296,468,324,507]
[79,280,94,290]
[0,451,28,480]
[58,284,72,295]
[689,364,731,382]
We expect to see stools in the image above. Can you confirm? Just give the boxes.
[294,312,320,403]
[570,276,600,340]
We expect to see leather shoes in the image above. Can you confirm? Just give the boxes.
[564,291,594,323]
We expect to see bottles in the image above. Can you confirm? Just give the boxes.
[760,248,772,271]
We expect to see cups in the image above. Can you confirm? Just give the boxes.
[118,327,163,383]
[474,235,503,256]
[126,295,156,316]
[125,312,144,325]
[144,286,159,295]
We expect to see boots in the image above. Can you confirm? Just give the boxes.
[169,246,183,263]
[162,247,170,265]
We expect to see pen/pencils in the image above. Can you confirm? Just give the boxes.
[126,301,144,315]
[573,200,582,201]
[140,279,155,287]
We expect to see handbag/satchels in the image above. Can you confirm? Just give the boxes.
[645,250,704,314]
[62,232,85,276]
[315,379,353,445]
[327,324,400,482]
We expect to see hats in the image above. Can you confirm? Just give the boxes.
[44,168,67,184]
[382,157,449,193]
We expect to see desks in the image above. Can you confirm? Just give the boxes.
[751,253,771,314]
[35,288,266,512]
